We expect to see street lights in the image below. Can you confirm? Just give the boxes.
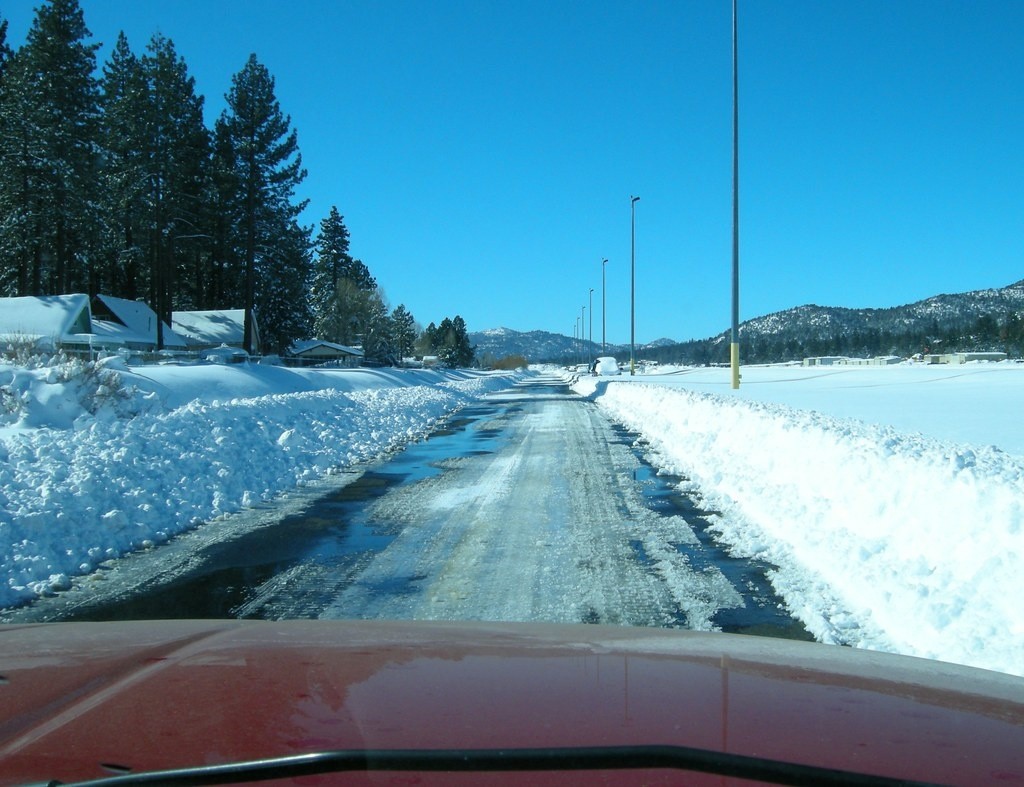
[576,317,580,364]
[632,196,645,375]
[582,306,586,364]
[602,259,611,356]
[573,325,576,366]
[589,289,594,367]
